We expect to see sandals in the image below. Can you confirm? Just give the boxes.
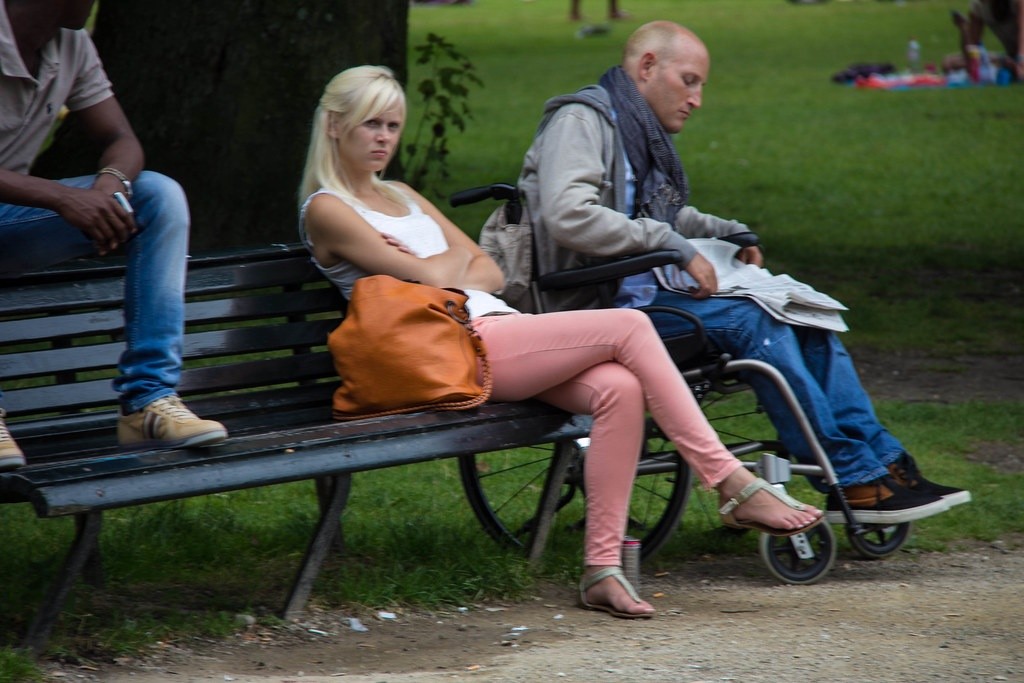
[577,566,656,620]
[718,478,825,537]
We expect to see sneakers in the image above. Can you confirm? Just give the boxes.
[0,409,28,469]
[115,394,227,452]
[884,453,972,508]
[827,468,950,523]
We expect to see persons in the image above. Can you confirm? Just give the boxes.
[516,20,974,525]
[300,66,823,617]
[940,0,1024,84]
[563,1,635,21]
[0,0,229,469]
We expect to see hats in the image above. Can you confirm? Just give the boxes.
[479,200,533,313]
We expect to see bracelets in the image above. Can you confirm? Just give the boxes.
[94,166,134,200]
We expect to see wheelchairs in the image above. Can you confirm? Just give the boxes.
[448,183,910,585]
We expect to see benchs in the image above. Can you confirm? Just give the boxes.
[0,241,593,650]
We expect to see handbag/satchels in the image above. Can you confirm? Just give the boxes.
[328,273,494,420]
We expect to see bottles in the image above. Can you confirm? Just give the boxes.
[907,39,921,62]
